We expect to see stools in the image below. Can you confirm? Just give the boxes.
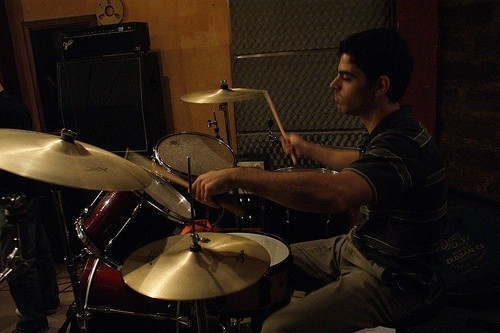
[382,281,444,333]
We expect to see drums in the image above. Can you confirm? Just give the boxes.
[128,151,189,201]
[204,230,294,326]
[78,257,177,333]
[261,167,359,246]
[72,163,195,271]
[146,131,239,182]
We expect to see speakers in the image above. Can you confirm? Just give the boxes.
[57,49,162,158]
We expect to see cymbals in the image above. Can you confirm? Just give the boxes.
[0,126,151,191]
[122,231,271,301]
[181,86,261,104]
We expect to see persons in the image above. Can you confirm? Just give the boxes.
[188,26,448,333]
[0,83,60,333]
